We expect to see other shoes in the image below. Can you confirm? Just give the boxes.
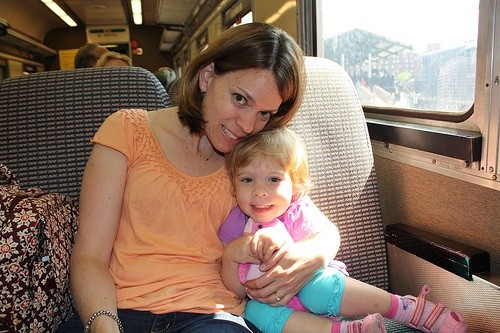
[403,285,468,333]
[362,313,387,333]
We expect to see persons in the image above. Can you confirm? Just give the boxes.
[153,66,177,90]
[95,51,132,68]
[54,21,313,333]
[217,125,470,333]
[74,42,108,70]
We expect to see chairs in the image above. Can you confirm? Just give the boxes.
[0,56,424,333]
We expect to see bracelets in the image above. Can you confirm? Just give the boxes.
[82,310,125,333]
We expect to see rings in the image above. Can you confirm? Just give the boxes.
[273,291,282,304]
